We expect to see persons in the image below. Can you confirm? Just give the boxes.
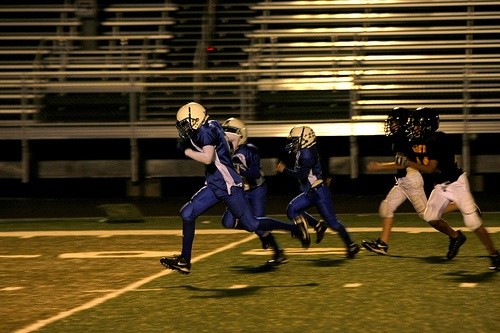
[361,106,500,271]
[160,102,311,274]
[219,118,288,267]
[275,126,360,258]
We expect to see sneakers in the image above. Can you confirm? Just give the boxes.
[474,202,482,216]
[314,220,328,244]
[492,254,500,270]
[447,230,466,260]
[362,241,388,255]
[347,243,359,257]
[160,256,191,275]
[293,214,310,247]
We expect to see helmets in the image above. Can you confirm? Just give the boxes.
[287,126,317,153]
[384,107,413,137]
[221,118,247,146]
[405,106,439,142]
[175,102,209,142]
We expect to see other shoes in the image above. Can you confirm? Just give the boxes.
[268,255,287,264]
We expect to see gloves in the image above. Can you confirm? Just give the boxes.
[396,154,409,169]
